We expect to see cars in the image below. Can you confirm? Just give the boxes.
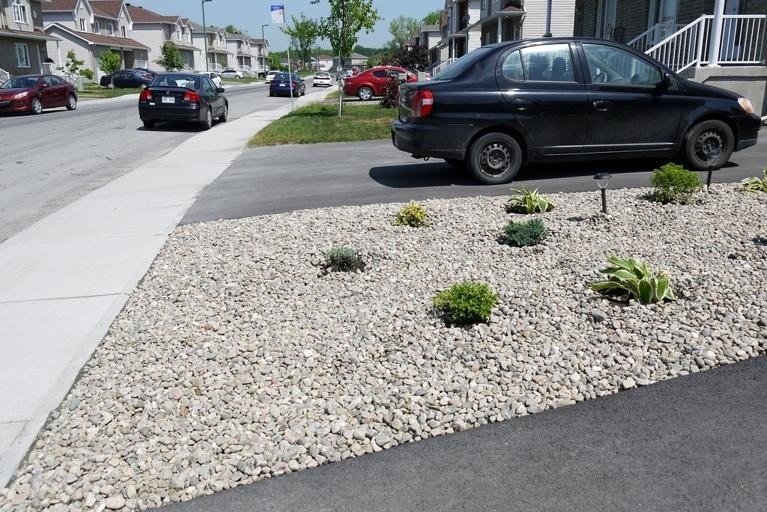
[212,69,244,80]
[0,74,78,117]
[313,71,333,88]
[269,73,306,97]
[198,73,222,88]
[100,69,152,90]
[255,69,268,78]
[265,69,281,83]
[334,67,361,80]
[134,67,157,76]
[138,72,228,131]
[392,37,760,186]
[343,65,433,100]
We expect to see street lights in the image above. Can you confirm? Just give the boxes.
[262,23,269,75]
[202,0,212,72]
